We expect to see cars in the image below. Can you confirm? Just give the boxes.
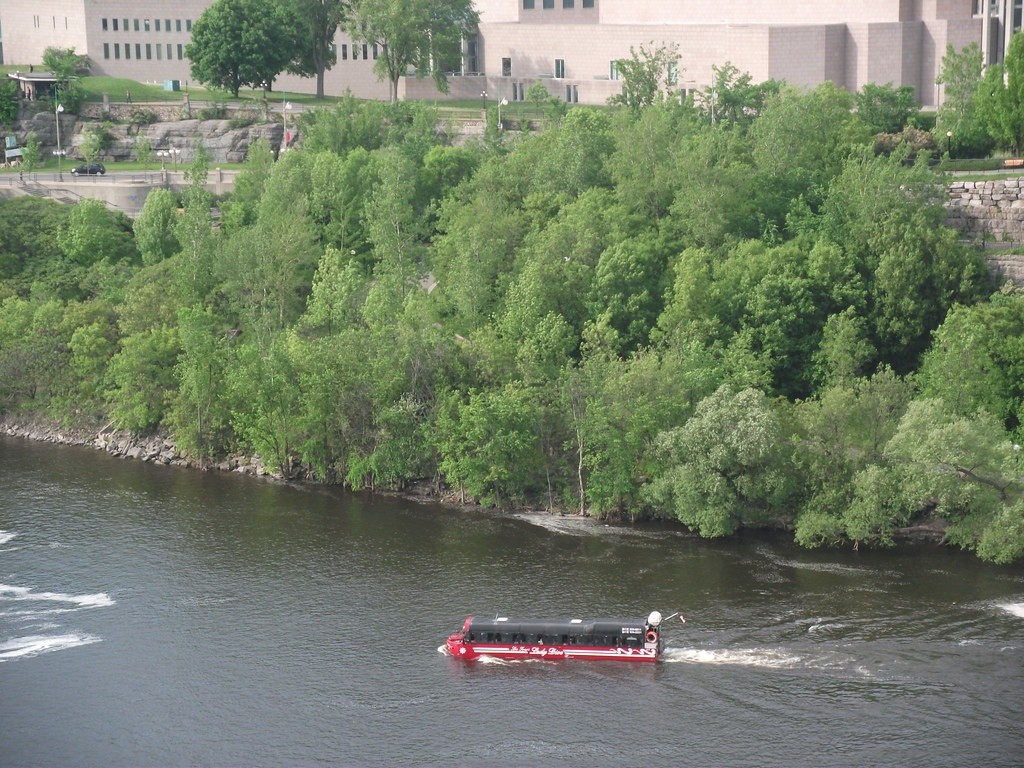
[71,163,105,177]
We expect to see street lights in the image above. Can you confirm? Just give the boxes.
[53,151,67,182]
[56,103,64,150]
[480,90,488,108]
[168,148,181,173]
[157,150,168,172]
[498,98,508,124]
[712,90,718,123]
[947,129,952,153]
[283,101,292,149]
[260,81,268,98]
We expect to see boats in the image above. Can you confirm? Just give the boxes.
[445,611,664,662]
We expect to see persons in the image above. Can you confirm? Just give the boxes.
[126,90,132,103]
[29,64,33,73]
[27,86,31,100]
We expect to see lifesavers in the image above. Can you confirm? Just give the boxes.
[646,631,657,643]
